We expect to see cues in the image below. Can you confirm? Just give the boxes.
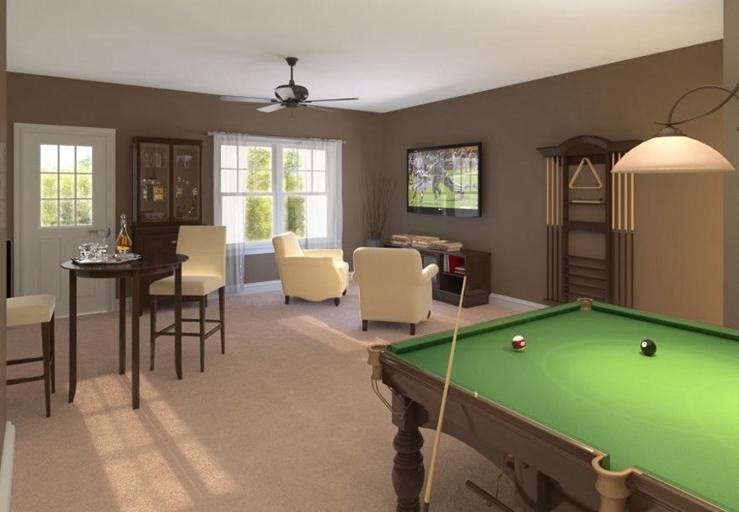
[625,173,627,307]
[612,152,614,304]
[551,156,553,300]
[423,276,467,511]
[556,157,558,303]
[631,172,634,307]
[560,156,563,302]
[466,480,515,511]
[617,152,620,306]
[546,157,549,300]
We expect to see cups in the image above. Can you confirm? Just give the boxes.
[79,242,107,262]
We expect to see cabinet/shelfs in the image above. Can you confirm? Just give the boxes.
[132,135,208,315]
[384,242,492,308]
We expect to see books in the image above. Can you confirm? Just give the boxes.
[391,232,463,251]
[439,253,465,275]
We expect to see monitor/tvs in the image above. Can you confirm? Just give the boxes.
[406,141,482,218]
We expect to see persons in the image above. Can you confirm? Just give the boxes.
[411,155,464,203]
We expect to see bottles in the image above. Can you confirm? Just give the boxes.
[143,153,198,223]
[115,215,133,259]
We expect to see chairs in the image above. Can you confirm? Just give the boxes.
[149,224,227,372]
[272,231,350,306]
[6,294,55,417]
[352,247,440,335]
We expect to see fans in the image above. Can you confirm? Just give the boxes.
[218,56,358,113]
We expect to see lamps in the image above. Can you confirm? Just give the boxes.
[611,82,739,173]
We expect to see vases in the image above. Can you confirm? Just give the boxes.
[366,239,384,247]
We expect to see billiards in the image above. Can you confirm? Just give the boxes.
[512,335,526,351]
[641,340,656,354]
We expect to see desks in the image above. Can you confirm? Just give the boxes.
[60,254,189,410]
[367,297,739,512]
